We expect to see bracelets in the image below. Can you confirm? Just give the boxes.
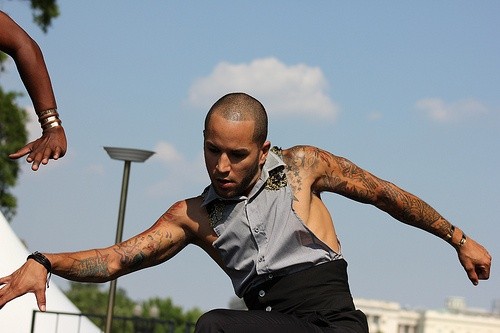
[27,251,52,275]
[38,109,61,129]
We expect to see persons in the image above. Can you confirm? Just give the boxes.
[0,92,491,333]
[0,10,68,170]
